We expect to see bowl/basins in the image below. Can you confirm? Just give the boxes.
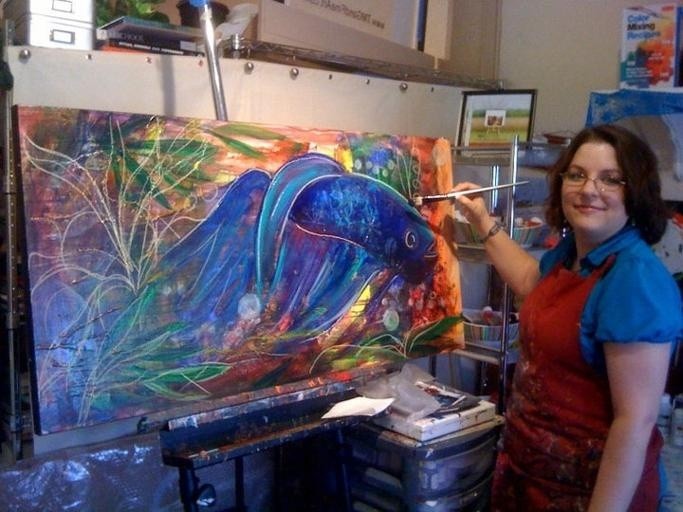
[453,215,545,250]
[461,310,519,349]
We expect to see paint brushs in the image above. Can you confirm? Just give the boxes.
[408,180,531,207]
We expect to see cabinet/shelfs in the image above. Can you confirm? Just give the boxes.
[427,143,564,416]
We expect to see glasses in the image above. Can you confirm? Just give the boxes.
[559,168,625,192]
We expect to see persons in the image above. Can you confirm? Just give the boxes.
[448,124,683,512]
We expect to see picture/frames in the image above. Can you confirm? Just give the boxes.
[453,89,536,154]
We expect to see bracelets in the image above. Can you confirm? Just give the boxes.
[479,221,502,245]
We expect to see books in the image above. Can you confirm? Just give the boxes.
[96,14,204,56]
